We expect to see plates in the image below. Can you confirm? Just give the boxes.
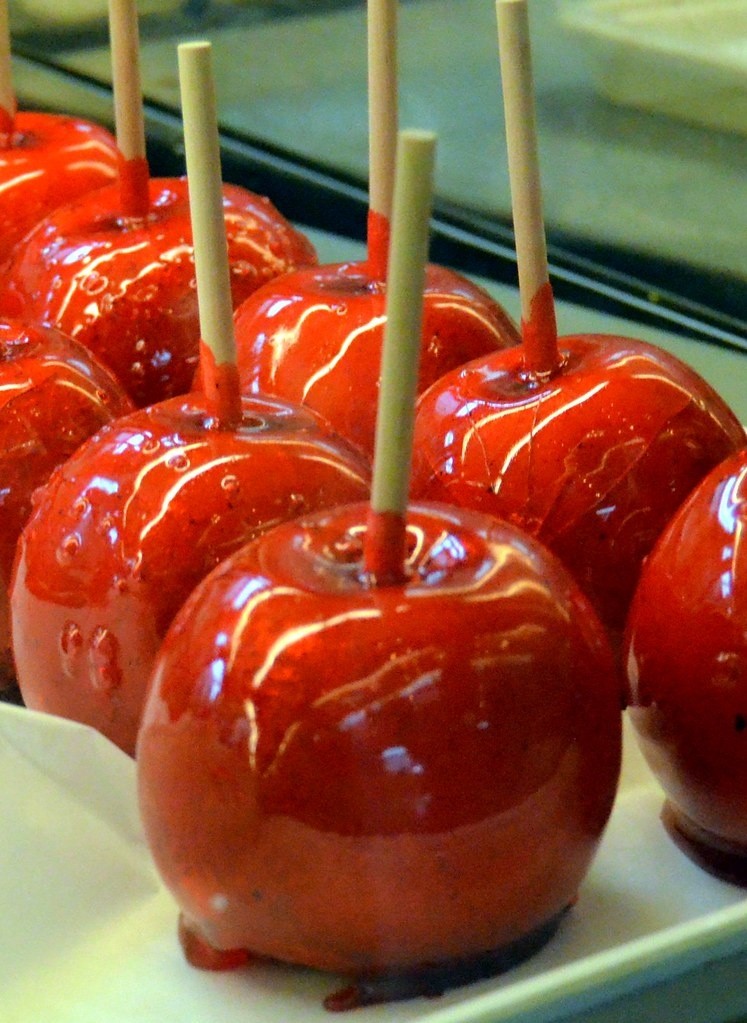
[3,227,746,1023]
[556,0,746,131]
